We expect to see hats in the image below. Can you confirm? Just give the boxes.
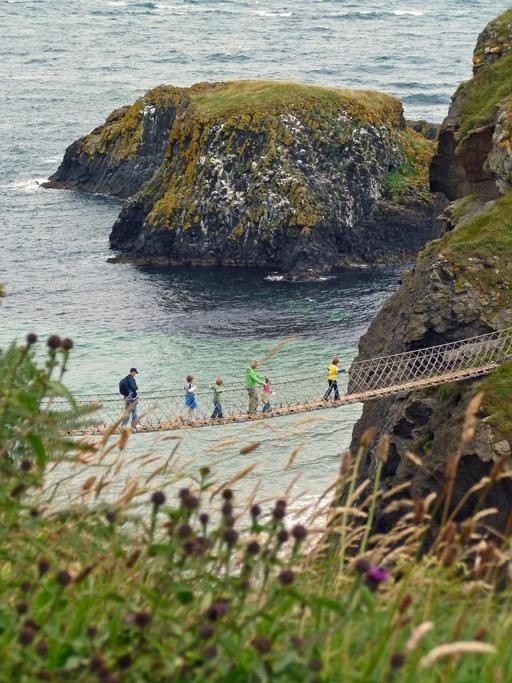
[129,367,137,374]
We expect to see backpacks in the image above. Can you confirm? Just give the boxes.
[118,375,133,396]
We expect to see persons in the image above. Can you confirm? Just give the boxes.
[319,357,347,401]
[120,366,140,428]
[179,374,199,424]
[260,376,271,412]
[243,359,267,413]
[208,377,225,419]
